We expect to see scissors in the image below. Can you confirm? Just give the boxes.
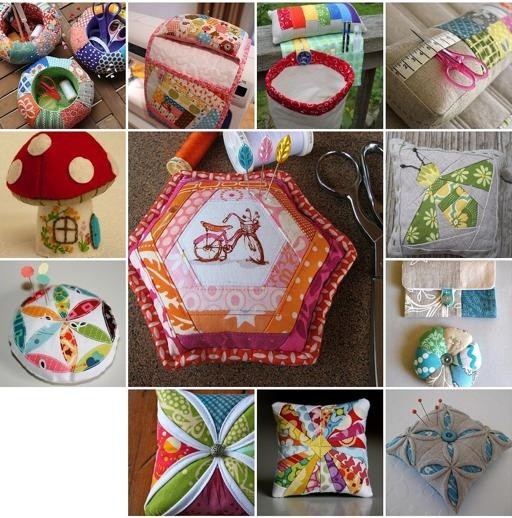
[93,2,126,47]
[410,29,489,91]
[36,75,66,105]
[316,141,383,387]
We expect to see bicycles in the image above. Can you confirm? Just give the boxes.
[194,207,265,264]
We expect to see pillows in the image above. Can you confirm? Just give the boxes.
[130,168,358,368]
[11,283,118,384]
[387,402,510,515]
[267,2,367,44]
[387,139,501,258]
[412,326,481,386]
[142,390,253,514]
[271,398,376,497]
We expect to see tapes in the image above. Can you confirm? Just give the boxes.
[88,36,111,53]
